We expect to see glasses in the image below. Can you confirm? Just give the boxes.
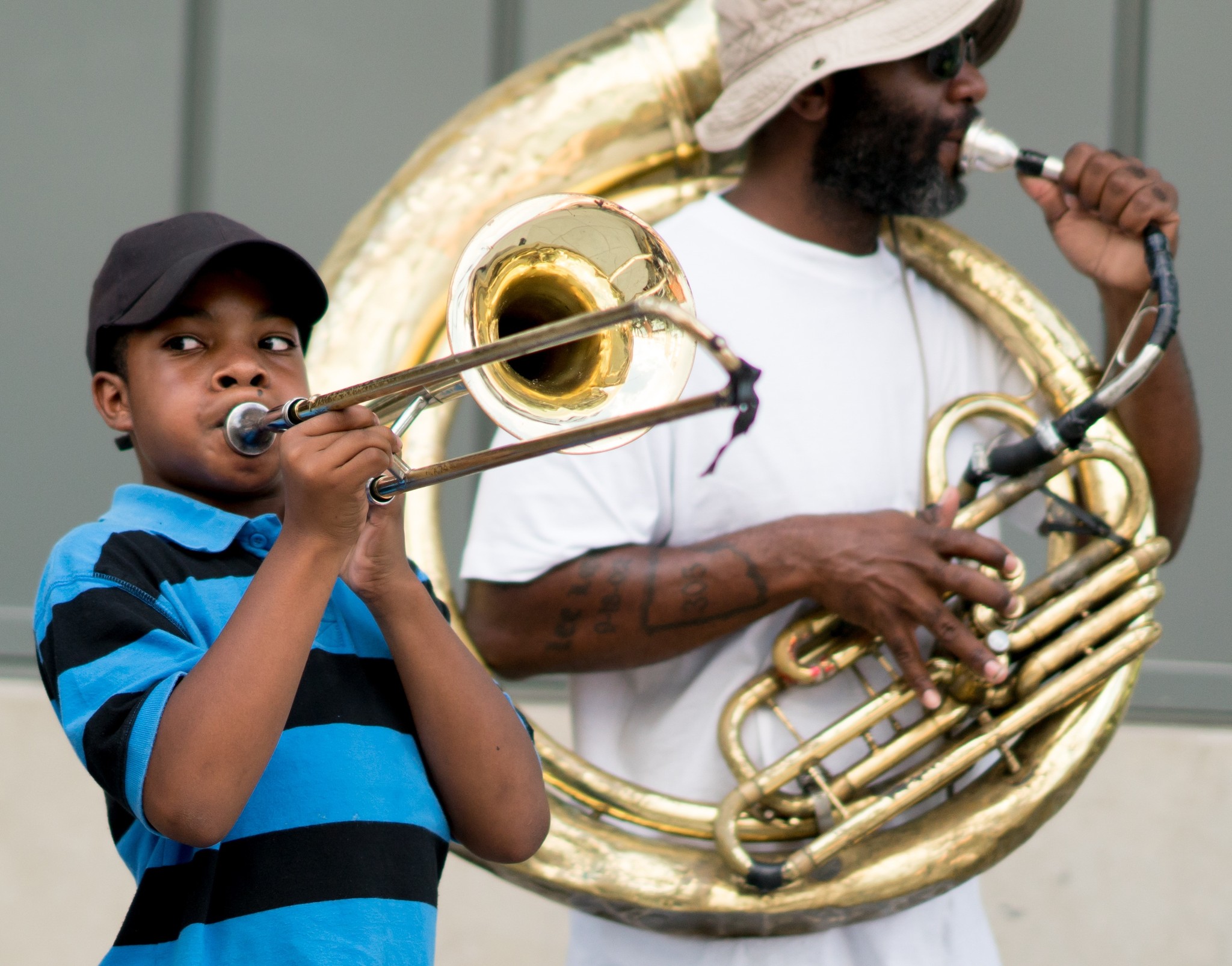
[909,34,979,78]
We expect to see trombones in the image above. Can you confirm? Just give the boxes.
[224,190,762,515]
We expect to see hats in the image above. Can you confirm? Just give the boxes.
[692,0,1023,157]
[84,211,328,452]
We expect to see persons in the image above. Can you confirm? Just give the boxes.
[460,1,1200,966]
[32,213,551,965]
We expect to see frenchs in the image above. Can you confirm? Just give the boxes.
[264,1,1181,938]
